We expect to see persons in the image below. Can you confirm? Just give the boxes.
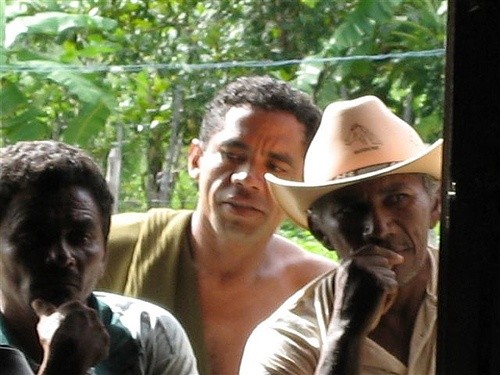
[0,140,199,375]
[238,94,444,375]
[94,76,340,375]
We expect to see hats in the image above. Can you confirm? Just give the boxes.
[262,95,441,228]
